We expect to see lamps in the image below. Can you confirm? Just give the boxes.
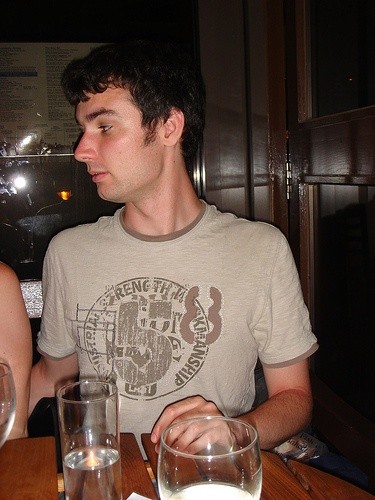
[20,190,72,264]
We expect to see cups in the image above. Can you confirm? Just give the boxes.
[156,414,263,500]
[170,478,252,500]
[0,363,17,449]
[57,379,123,500]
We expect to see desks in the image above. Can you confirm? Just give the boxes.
[0,433,375,500]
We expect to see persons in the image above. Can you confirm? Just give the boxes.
[26,40,320,459]
[0,262,32,441]
